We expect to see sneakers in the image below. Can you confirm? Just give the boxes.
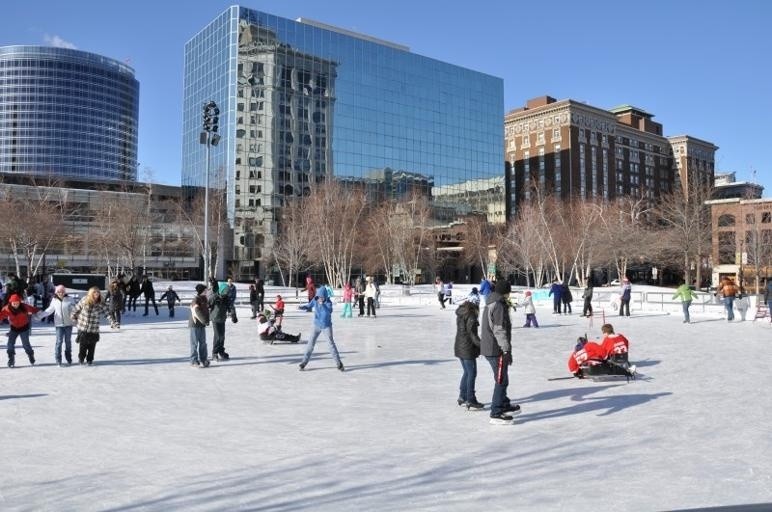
[8,357,93,366]
[729,317,735,321]
[683,320,691,323]
[251,316,257,319]
[551,312,593,318]
[627,314,631,316]
[341,314,376,319]
[619,313,624,316]
[339,365,344,372]
[190,352,229,368]
[300,362,307,370]
[123,308,175,319]
[534,325,539,328]
[523,324,530,327]
[440,306,445,309]
[458,397,520,420]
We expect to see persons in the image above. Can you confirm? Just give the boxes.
[434,275,494,311]
[713,275,741,321]
[566,336,637,380]
[478,280,520,421]
[601,324,628,364]
[324,281,333,301]
[548,279,562,313]
[249,277,302,343]
[453,293,485,409]
[519,290,540,328]
[341,275,381,318]
[618,282,634,316]
[301,276,316,313]
[188,276,238,369]
[763,276,772,324]
[671,278,698,324]
[558,279,572,314]
[104,273,159,329]
[0,270,120,365]
[581,280,593,316]
[298,287,344,372]
[158,285,180,319]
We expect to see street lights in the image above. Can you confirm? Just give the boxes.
[201,97,222,287]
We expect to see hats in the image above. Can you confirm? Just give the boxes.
[195,283,204,294]
[55,285,65,292]
[466,287,480,302]
[9,295,21,302]
[495,281,510,292]
